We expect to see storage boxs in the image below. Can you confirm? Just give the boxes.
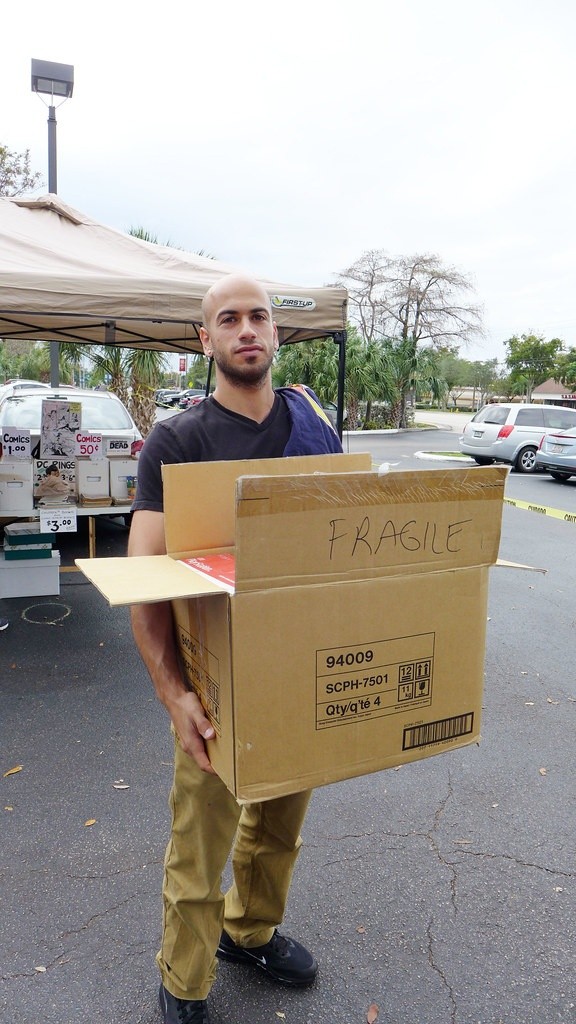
[2,537,52,560]
[1,455,34,511]
[0,550,60,599]
[73,453,513,807]
[75,455,110,503]
[4,522,55,546]
[105,455,139,497]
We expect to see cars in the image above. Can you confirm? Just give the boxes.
[155,384,211,410]
[534,424,576,481]
[0,379,145,466]
[458,400,576,472]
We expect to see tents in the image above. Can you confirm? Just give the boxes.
[0,192,349,354]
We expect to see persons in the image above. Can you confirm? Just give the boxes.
[125,271,346,1024]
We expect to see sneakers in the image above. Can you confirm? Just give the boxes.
[159,983,210,1024]
[215,927,318,986]
